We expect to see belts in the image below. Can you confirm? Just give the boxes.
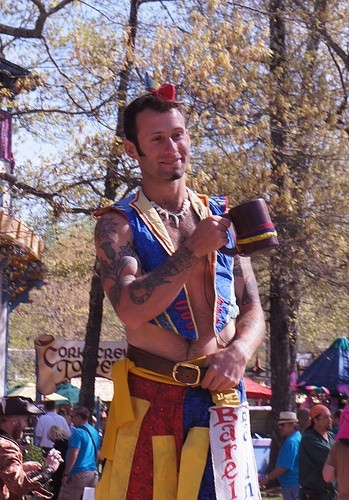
[124,344,209,386]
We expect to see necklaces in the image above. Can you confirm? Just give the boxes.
[151,199,191,229]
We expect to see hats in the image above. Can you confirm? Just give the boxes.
[334,405,349,440]
[0,395,45,415]
[310,405,331,417]
[146,83,184,106]
[277,412,299,424]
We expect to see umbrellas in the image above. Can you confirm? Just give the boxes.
[5,382,81,406]
[295,337,349,399]
[243,376,273,400]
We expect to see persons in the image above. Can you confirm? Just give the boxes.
[322,405,349,500]
[58,406,101,500]
[258,412,302,500]
[35,400,70,458]
[93,83,266,500]
[47,425,68,500]
[332,398,348,431]
[0,397,64,500]
[298,405,339,500]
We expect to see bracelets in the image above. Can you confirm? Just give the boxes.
[266,474,269,481]
[63,473,69,477]
[45,465,54,475]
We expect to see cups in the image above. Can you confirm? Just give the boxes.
[217,199,279,256]
